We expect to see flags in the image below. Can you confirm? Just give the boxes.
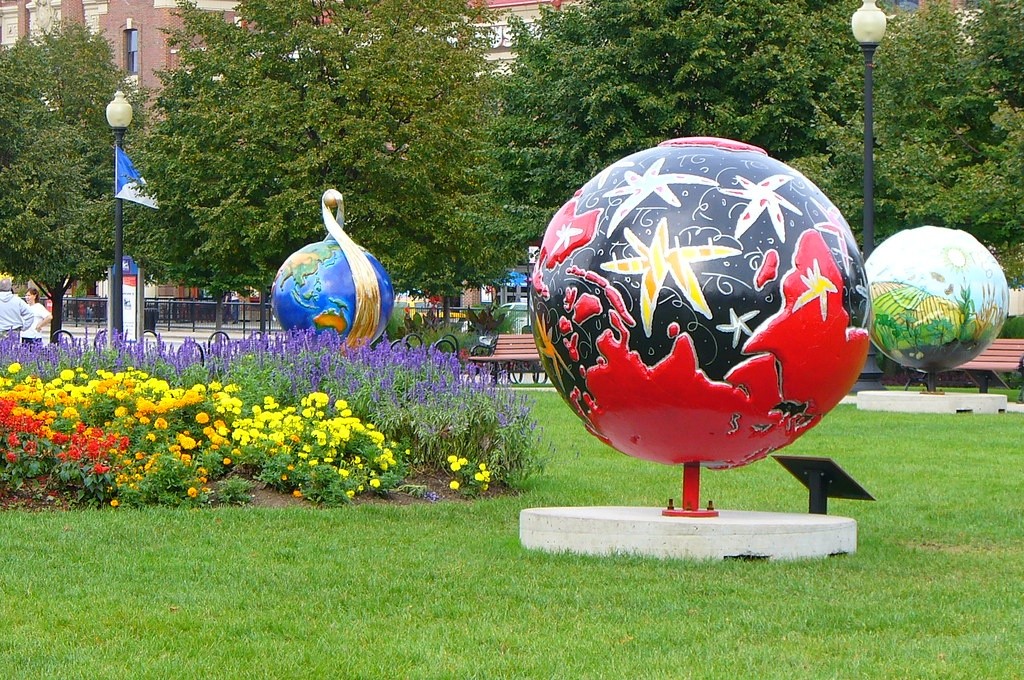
[116,147,158,209]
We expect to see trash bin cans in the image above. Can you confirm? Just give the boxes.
[144,308,157,332]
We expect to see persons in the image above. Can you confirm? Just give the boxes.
[0,278,34,341]
[21,288,53,352]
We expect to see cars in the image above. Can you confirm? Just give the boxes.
[462,301,533,335]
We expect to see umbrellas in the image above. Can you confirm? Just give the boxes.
[491,271,527,301]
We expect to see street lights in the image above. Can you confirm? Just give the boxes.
[105,90,132,348]
[851,0,888,262]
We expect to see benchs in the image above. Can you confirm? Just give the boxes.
[899,339,1024,399]
[467,332,548,384]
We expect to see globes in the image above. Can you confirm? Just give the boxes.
[271,189,393,357]
